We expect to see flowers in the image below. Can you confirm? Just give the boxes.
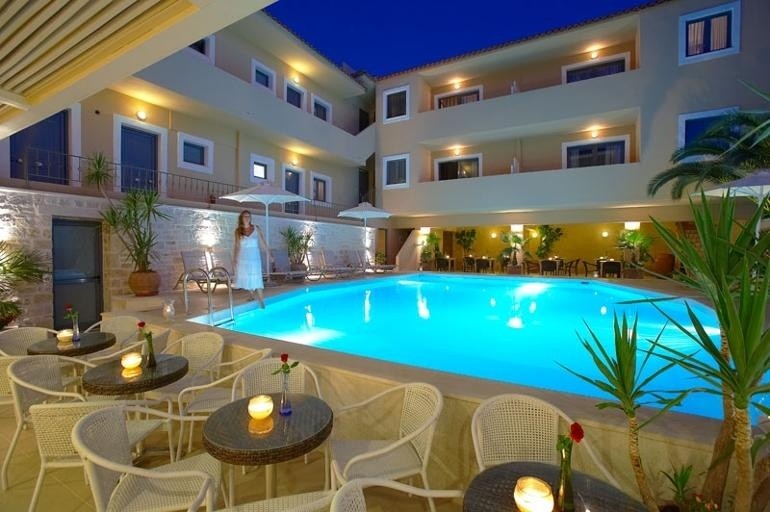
[569,421,586,444]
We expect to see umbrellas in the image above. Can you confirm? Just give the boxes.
[337,202,394,263]
[218,184,312,283]
[690,170,770,296]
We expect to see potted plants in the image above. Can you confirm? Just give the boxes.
[89,147,175,297]
[616,225,657,282]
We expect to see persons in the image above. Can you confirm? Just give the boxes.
[232,210,274,309]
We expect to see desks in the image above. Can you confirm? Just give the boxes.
[459,458,656,512]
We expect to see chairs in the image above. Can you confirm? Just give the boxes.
[1,304,444,511]
[424,249,626,278]
[329,478,462,512]
[469,391,580,472]
[170,243,400,296]
